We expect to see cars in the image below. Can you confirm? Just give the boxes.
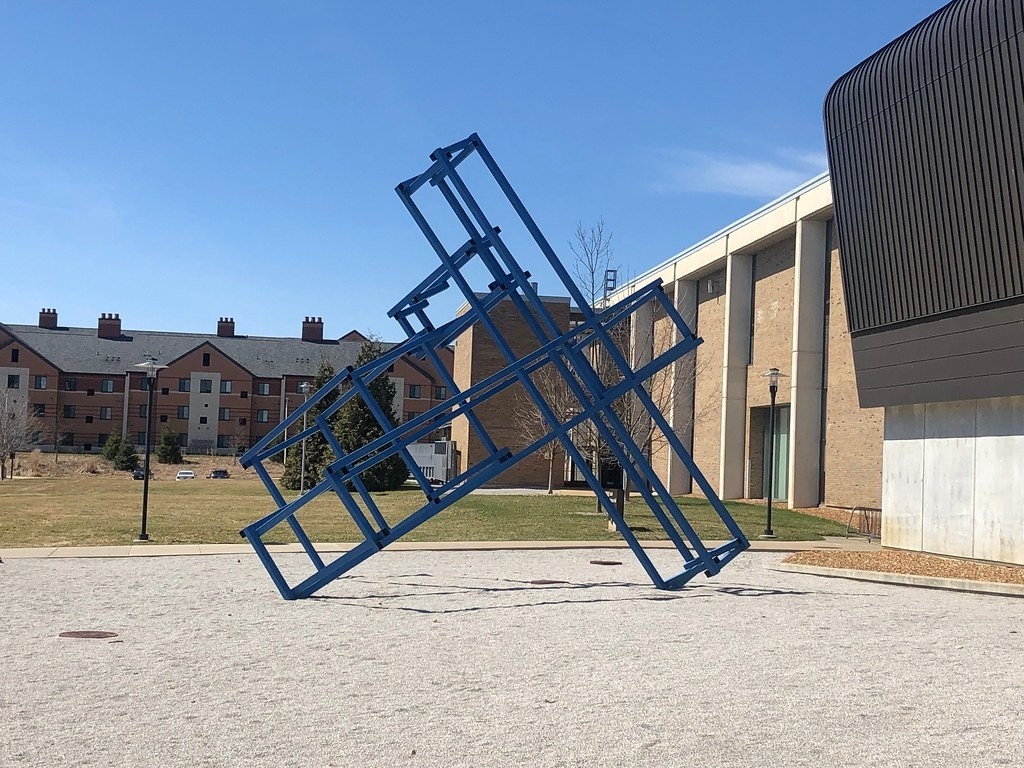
[133,468,154,480]
[209,468,231,479]
[175,470,197,481]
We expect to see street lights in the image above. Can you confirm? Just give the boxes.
[130,357,170,542]
[758,368,789,538]
[283,397,291,465]
[298,381,316,499]
